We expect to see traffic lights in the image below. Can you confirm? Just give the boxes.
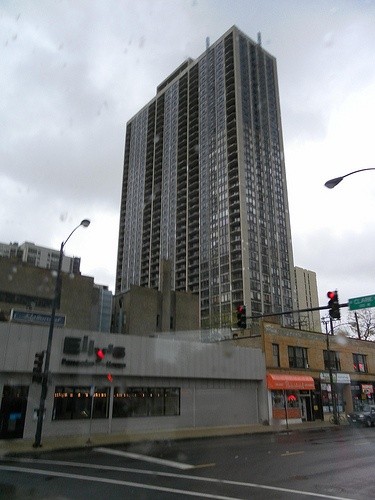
[327,289,341,319]
[236,305,247,329]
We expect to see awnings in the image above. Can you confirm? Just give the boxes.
[267,374,315,391]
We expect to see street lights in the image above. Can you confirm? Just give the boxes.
[32,219,92,449]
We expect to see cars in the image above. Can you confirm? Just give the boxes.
[349,405,375,427]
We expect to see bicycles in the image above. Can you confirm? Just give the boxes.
[329,414,345,425]
